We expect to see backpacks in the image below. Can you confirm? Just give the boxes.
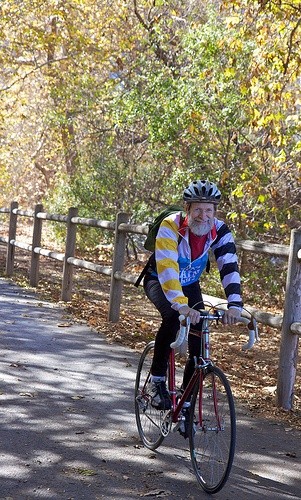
[133,206,209,288]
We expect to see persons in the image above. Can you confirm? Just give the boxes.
[142,178,245,437]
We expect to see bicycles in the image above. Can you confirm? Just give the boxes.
[134,301,260,494]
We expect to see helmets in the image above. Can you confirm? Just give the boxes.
[181,180,221,205]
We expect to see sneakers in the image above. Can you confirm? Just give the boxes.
[145,378,171,411]
[172,395,196,440]
